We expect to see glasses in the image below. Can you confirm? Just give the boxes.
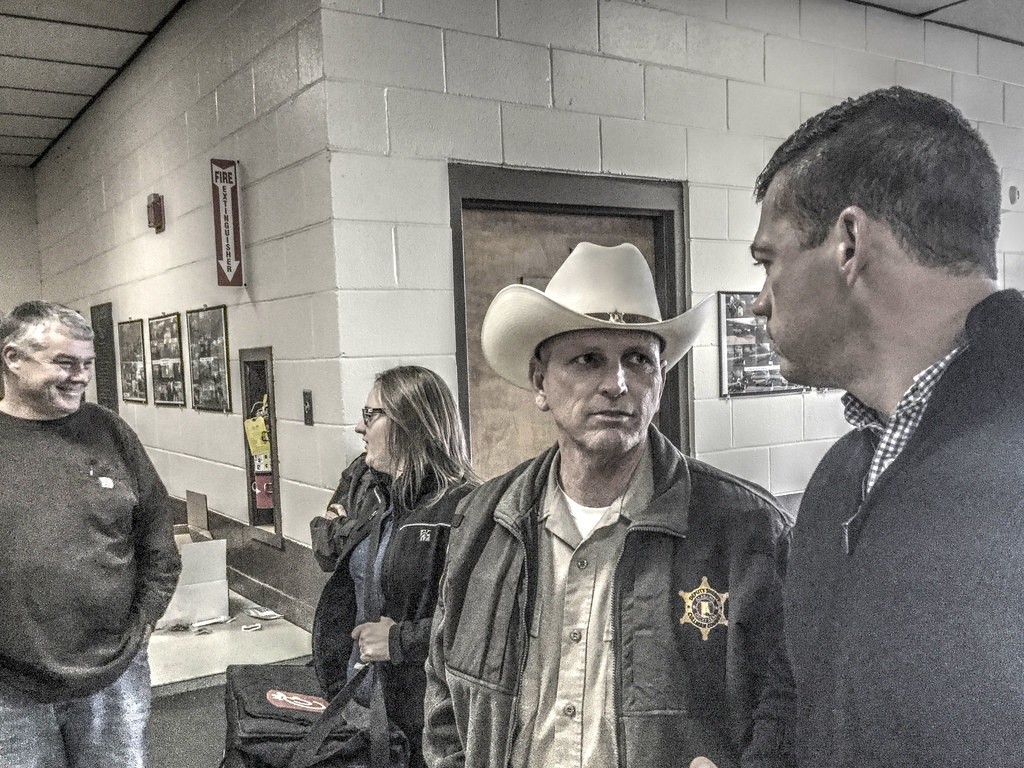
[362,407,387,427]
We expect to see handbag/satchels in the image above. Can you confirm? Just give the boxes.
[219,664,409,768]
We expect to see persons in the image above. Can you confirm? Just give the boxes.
[309,364,487,768]
[0,299,183,768]
[736,85,1024,768]
[421,242,796,768]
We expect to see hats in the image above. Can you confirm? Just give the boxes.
[481,242,716,392]
